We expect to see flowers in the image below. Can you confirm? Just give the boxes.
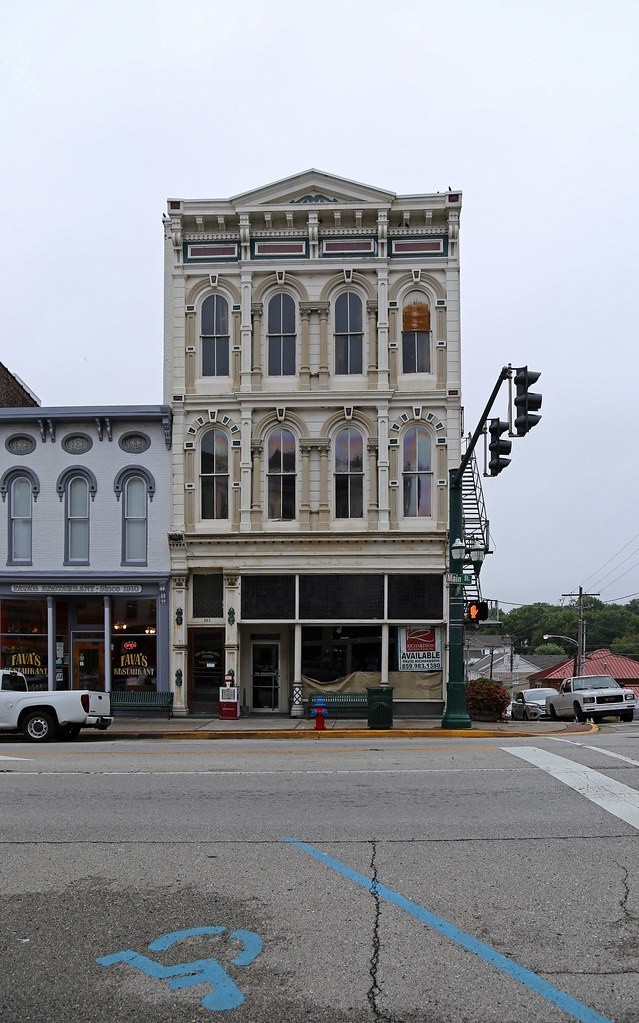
[465,677,512,723]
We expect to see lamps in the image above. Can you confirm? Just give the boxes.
[145,626,156,634]
[114,621,127,629]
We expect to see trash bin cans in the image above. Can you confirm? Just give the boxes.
[364,685,396,729]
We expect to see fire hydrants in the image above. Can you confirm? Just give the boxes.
[311,696,329,730]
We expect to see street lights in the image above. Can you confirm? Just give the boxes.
[441,537,486,729]
[544,635,583,676]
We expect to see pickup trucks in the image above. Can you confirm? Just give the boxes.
[544,675,635,723]
[0,670,113,742]
[78,666,157,692]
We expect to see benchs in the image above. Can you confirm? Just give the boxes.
[307,691,368,721]
[108,691,174,720]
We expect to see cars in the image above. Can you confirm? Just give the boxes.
[511,688,561,721]
[506,700,517,719]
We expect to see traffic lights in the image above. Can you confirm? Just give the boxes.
[467,602,488,621]
[488,417,512,475]
[514,364,541,435]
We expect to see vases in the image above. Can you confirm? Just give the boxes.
[467,707,501,722]
